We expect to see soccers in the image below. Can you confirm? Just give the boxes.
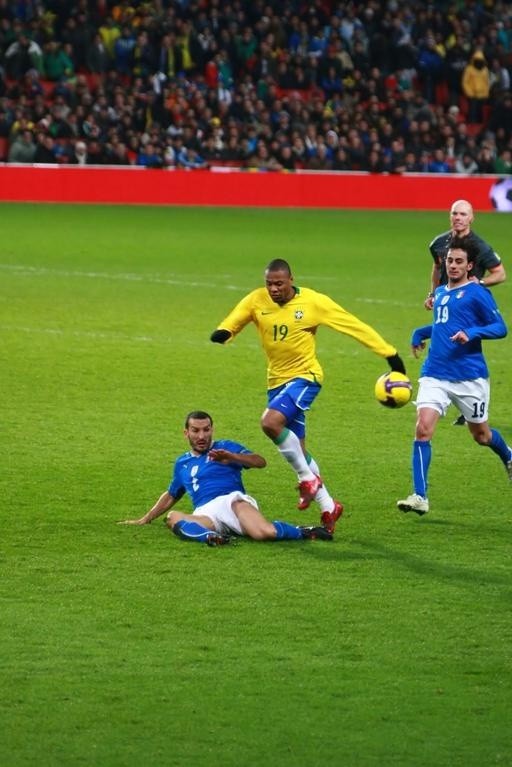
[374,370,412,407]
[490,176,512,213]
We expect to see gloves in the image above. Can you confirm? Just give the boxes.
[386,354,405,376]
[211,331,231,343]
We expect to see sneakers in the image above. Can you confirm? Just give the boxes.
[505,446,512,479]
[207,533,230,547]
[296,526,333,540]
[397,494,429,515]
[453,414,468,425]
[298,474,322,510]
[320,503,343,533]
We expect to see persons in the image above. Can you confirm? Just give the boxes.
[423,199,508,426]
[396,235,511,516]
[210,258,407,537]
[0,1,511,175]
[116,411,334,548]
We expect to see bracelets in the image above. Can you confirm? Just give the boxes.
[428,292,434,298]
[479,278,485,287]
